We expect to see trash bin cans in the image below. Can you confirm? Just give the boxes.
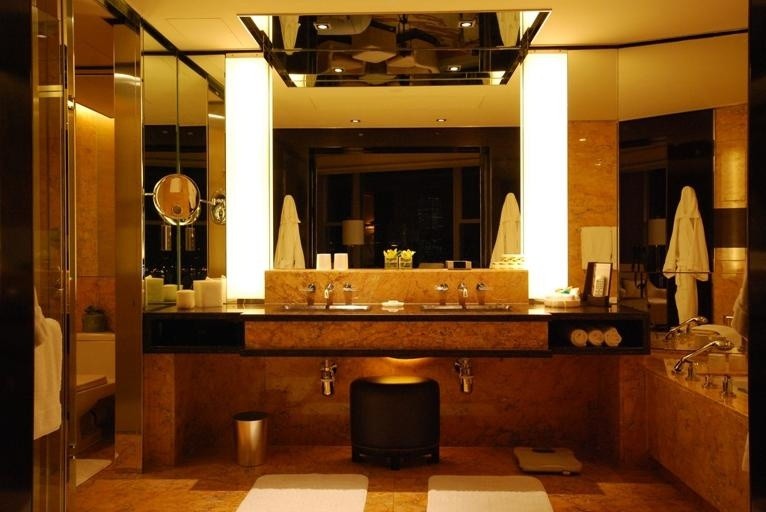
[233,411,269,467]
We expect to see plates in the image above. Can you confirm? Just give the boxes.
[545,300,582,307]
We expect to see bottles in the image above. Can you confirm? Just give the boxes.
[163,284,176,303]
[176,289,195,309]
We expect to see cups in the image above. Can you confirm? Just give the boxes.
[317,253,349,269]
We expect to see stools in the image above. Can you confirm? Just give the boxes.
[349,376,440,471]
[235,474,368,512]
[426,475,554,512]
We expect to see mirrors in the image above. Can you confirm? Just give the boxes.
[615,108,716,333]
[140,54,227,291]
[274,70,520,268]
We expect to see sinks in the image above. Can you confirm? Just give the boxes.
[420,303,518,312]
[277,304,369,311]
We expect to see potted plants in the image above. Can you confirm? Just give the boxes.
[383,249,416,270]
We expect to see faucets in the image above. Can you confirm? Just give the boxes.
[673,338,734,372]
[664,316,708,339]
[458,283,468,297]
[324,285,333,299]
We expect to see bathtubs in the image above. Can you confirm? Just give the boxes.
[731,375,749,400]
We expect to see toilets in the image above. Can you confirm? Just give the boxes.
[75,332,116,454]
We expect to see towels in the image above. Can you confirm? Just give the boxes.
[34,318,63,442]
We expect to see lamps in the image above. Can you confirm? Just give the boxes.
[154,56,200,220]
[647,219,667,272]
[342,221,364,247]
